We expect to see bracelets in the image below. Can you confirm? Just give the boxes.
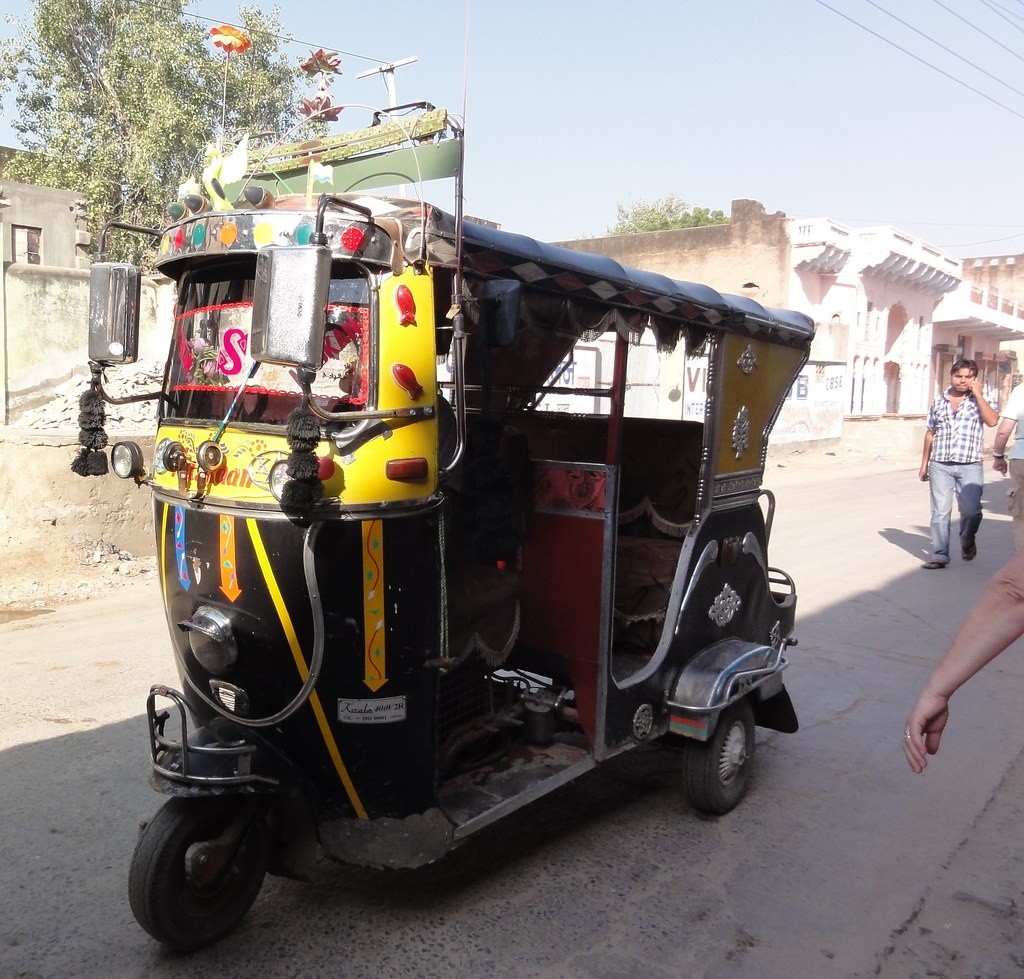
[993,451,1003,456]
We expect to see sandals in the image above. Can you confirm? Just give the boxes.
[921,558,948,569]
[961,537,976,560]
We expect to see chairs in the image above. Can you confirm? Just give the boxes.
[409,410,530,665]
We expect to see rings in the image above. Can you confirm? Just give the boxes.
[904,729,910,737]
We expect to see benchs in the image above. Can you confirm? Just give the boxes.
[504,410,705,656]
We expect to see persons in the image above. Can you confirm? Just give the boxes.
[899,544,1024,772]
[991,377,1024,544]
[918,358,1000,569]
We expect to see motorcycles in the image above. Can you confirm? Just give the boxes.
[86,98,817,955]
